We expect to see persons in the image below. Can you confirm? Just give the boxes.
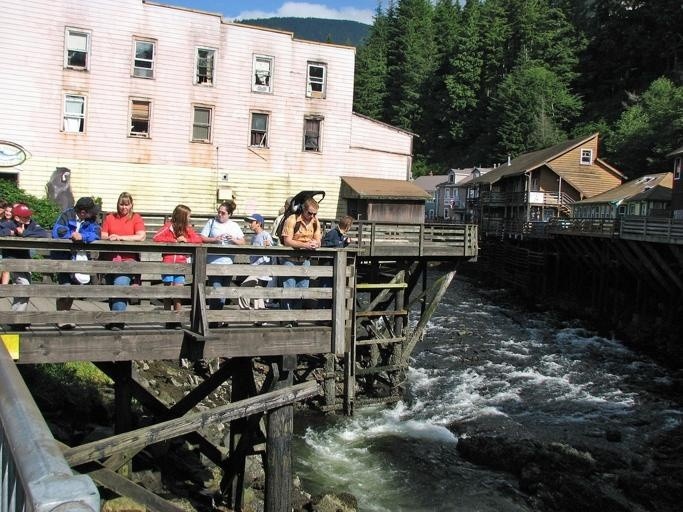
[0,199,7,218]
[153,205,202,328]
[239,213,273,326]
[99,192,146,331]
[52,196,100,329]
[317,215,352,308]
[0,205,48,326]
[264,197,295,307]
[281,198,320,327]
[200,201,245,328]
[0,206,13,284]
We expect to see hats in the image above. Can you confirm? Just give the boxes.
[12,204,33,217]
[244,214,264,224]
[76,197,101,215]
